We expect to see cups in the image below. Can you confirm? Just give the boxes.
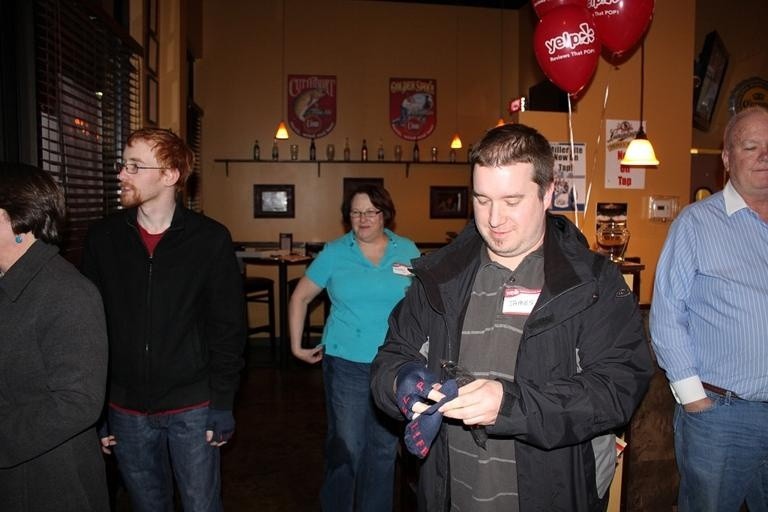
[326,144,335,161]
[394,145,402,160]
[280,233,293,255]
[290,144,299,160]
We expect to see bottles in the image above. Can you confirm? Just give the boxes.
[361,139,368,161]
[254,140,260,160]
[343,137,350,161]
[413,139,420,162]
[271,137,279,161]
[431,147,438,161]
[377,136,385,160]
[450,149,456,164]
[309,137,317,161]
[467,144,473,163]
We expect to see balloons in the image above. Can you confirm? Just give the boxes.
[533,5,600,93]
[586,0,654,58]
[532,0,587,17]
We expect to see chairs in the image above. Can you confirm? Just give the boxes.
[288,240,330,349]
[231,240,276,348]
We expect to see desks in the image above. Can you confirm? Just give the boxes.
[241,251,313,356]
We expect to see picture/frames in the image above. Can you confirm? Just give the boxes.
[342,177,384,199]
[429,184,470,220]
[252,183,296,220]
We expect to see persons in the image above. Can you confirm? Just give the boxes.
[370,125,656,512]
[649,107,768,512]
[287,185,422,512]
[79,127,248,512]
[0,161,111,512]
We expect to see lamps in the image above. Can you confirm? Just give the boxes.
[617,42,661,168]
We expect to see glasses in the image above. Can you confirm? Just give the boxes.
[348,209,382,219]
[113,162,165,174]
